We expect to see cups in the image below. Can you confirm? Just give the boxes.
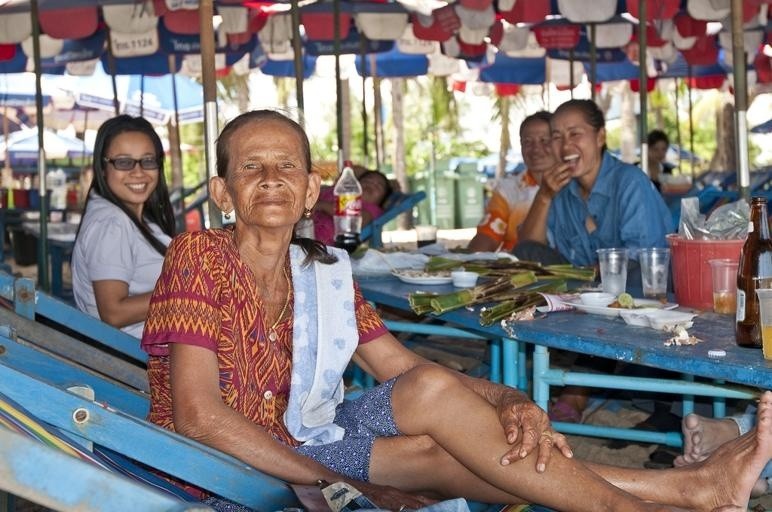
[103,157,160,170]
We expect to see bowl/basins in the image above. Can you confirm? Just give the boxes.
[268,265,291,332]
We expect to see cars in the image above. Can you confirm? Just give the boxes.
[596,248,629,296]
[415,225,437,248]
[637,247,671,298]
[709,259,740,315]
[451,272,479,288]
[755,287,772,362]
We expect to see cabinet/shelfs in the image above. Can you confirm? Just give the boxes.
[735,196,772,349]
[333,160,363,253]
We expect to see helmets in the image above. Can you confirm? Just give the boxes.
[391,269,455,285]
[563,292,679,318]
[475,250,518,263]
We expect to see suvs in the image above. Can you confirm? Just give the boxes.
[663,232,755,309]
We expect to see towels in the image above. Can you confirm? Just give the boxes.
[409,169,431,226]
[429,159,455,230]
[455,161,485,229]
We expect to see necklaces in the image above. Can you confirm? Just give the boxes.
[268,265,291,332]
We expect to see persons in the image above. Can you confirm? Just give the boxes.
[68,114,178,340]
[451,99,678,424]
[630,130,690,184]
[508,99,678,424]
[295,160,400,249]
[674,401,772,499]
[141,106,771,512]
[141,110,771,512]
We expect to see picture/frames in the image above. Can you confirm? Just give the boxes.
[25,223,77,302]
[353,273,771,482]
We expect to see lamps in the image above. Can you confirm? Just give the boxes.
[358,191,428,254]
[0,271,553,512]
[707,164,772,231]
[667,169,735,231]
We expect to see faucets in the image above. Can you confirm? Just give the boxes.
[644,444,682,470]
